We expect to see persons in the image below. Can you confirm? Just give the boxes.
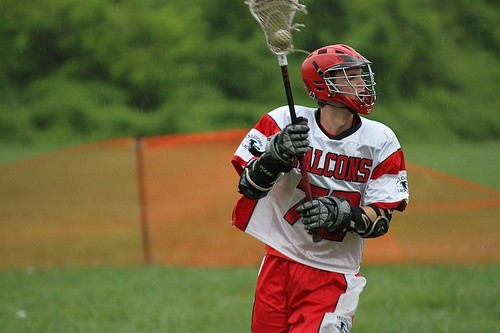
[229,44,409,333]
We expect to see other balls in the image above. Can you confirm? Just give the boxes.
[271,28,293,51]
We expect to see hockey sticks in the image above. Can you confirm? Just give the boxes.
[245,0,316,204]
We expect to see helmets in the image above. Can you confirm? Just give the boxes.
[304,42,372,77]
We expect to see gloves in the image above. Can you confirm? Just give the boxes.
[267,116,310,168]
[297,194,351,234]
